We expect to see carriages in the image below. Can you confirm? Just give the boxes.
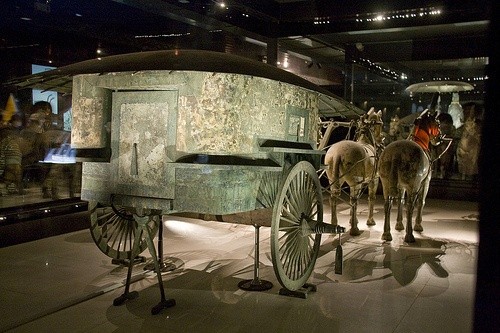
[50,71,443,292]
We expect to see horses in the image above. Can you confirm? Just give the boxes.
[325,105,444,245]
[0,106,81,201]
